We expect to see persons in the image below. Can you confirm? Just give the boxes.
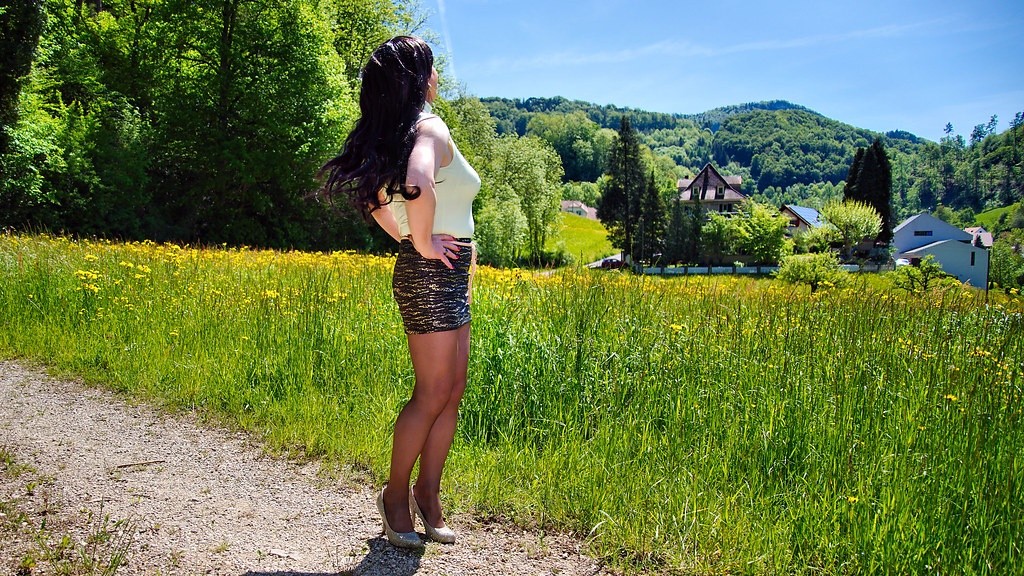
[315,36,483,548]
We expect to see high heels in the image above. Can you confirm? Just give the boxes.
[377,485,422,549]
[409,483,456,543]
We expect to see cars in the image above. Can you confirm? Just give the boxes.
[602,259,624,268]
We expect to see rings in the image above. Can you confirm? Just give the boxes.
[444,248,448,255]
